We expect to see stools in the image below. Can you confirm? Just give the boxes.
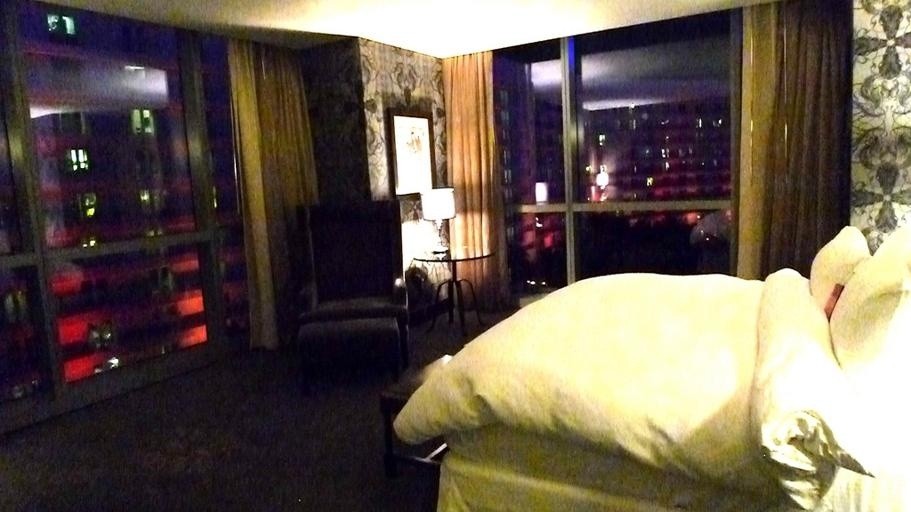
[379,352,453,481]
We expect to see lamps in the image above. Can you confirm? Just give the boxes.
[421,187,456,254]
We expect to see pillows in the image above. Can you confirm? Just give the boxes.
[828,225,911,376]
[810,226,871,322]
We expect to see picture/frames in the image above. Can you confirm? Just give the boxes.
[386,108,437,201]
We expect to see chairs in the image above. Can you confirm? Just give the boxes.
[285,199,410,393]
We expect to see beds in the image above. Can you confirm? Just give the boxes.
[437,274,911,512]
